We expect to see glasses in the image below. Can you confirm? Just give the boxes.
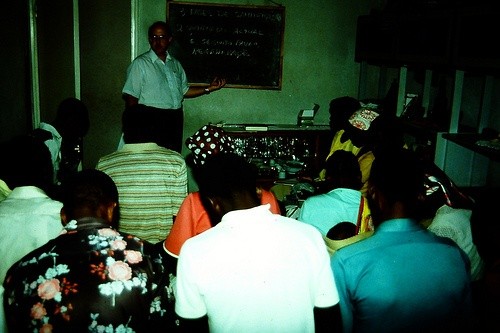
[149,34,167,39]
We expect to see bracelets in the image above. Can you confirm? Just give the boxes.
[205,89,210,94]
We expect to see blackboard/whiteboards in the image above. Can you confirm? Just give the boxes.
[165,1,286,91]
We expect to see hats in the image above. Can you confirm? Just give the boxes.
[185,125,234,165]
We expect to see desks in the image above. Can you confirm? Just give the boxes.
[216,122,321,184]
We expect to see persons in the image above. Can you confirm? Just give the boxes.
[0,143,67,287]
[174,156,347,333]
[330,154,471,333]
[94,104,189,244]
[158,124,280,272]
[297,149,361,241]
[2,168,203,333]
[38,95,90,187]
[121,21,226,154]
[421,188,500,333]
[315,97,376,197]
